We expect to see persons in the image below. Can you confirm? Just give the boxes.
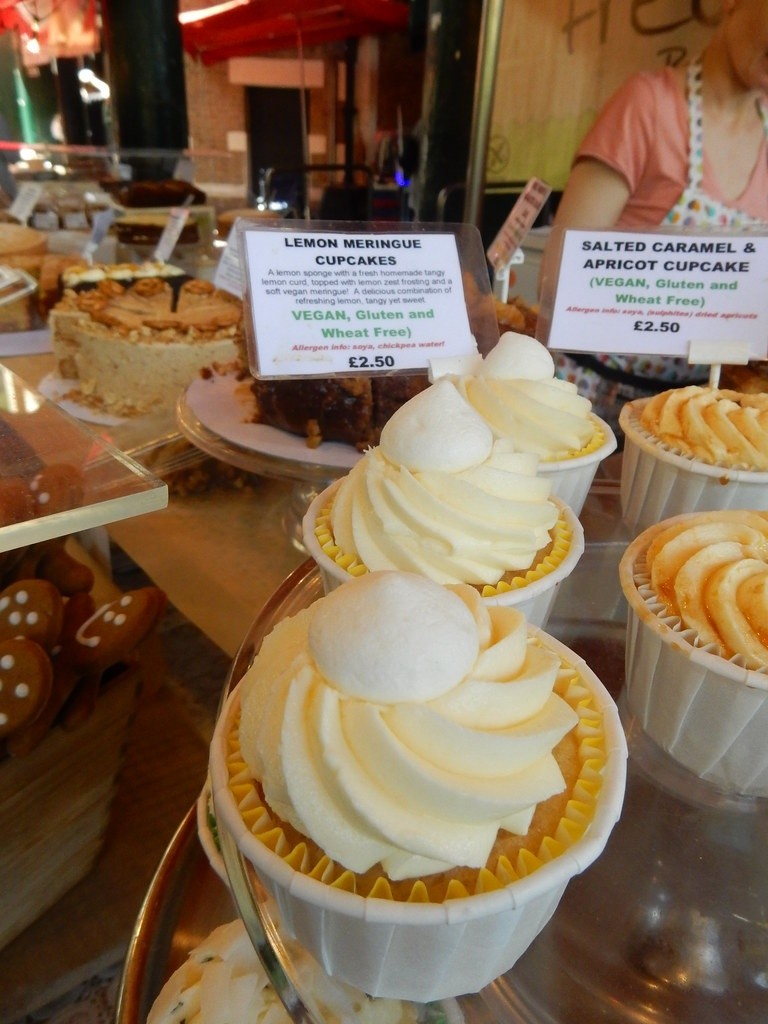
[534,0,768,439]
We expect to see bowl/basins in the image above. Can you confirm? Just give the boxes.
[536,411,617,515]
[305,475,585,630]
[621,511,768,799]
[211,626,627,1000]
[619,394,768,541]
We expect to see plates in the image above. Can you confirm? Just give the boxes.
[188,370,365,470]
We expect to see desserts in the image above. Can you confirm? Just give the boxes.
[0,173,768,1002]
